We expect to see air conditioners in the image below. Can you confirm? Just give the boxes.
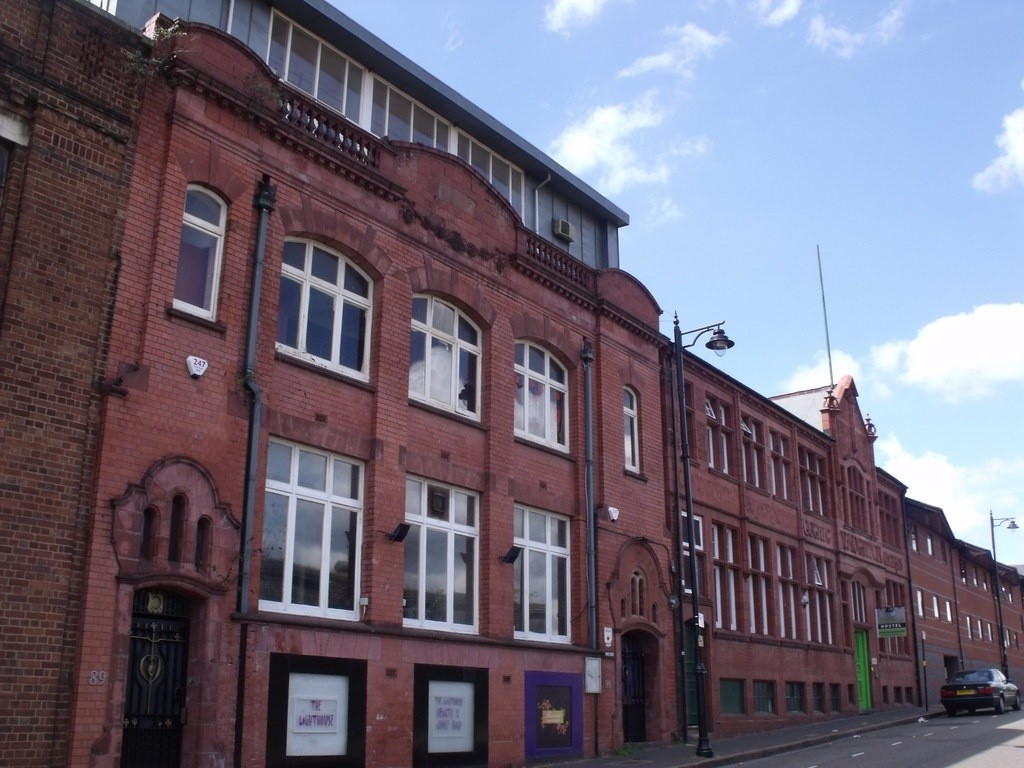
[555,219,576,242]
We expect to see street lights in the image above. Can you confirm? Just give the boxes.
[672,309,736,757]
[988,509,1020,678]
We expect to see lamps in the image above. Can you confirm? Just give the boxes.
[379,523,411,542]
[500,546,521,564]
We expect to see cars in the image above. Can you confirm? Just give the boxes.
[940,668,1021,718]
